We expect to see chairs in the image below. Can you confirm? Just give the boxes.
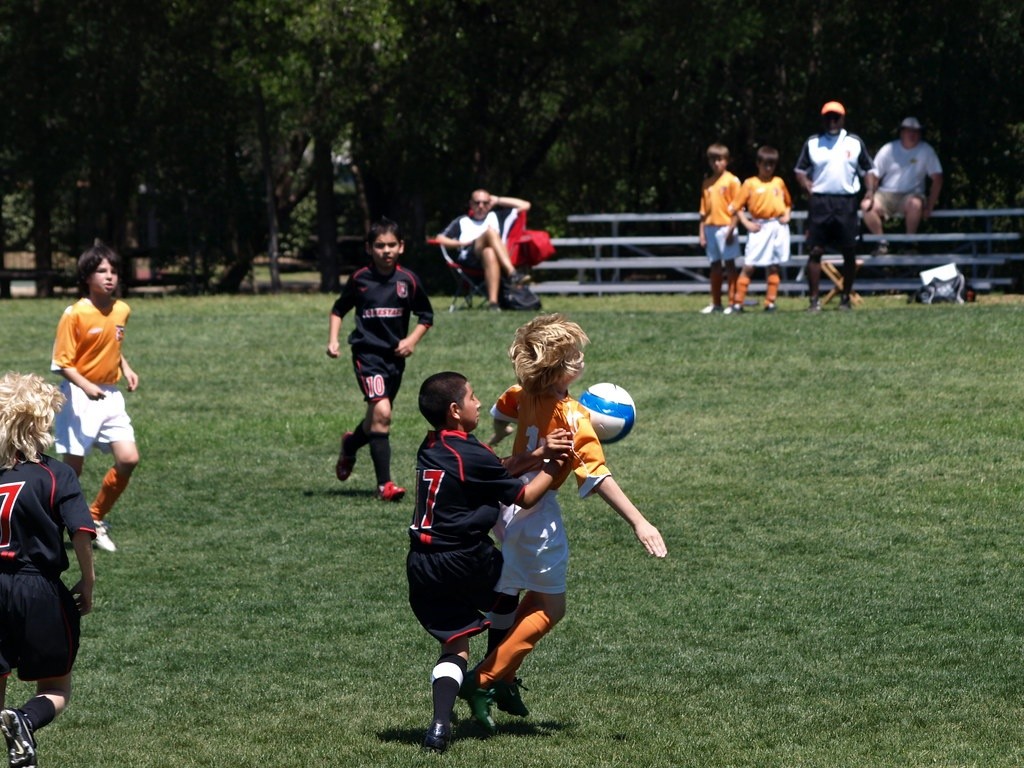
[438,211,527,313]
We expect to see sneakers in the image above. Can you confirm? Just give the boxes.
[336,432,356,481]
[0,708,37,768]
[455,667,496,736]
[486,678,530,719]
[90,520,115,552]
[377,481,404,502]
[425,720,450,750]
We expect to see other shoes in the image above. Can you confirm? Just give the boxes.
[838,294,850,312]
[765,304,777,313]
[807,300,820,315]
[486,305,501,314]
[723,303,742,315]
[510,271,530,283]
[700,304,723,315]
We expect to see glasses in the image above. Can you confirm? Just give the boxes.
[471,199,489,205]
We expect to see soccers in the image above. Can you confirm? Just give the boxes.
[578,382,637,444]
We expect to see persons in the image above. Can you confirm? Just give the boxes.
[726,146,793,312]
[436,190,531,311]
[457,312,668,735]
[698,143,742,314]
[327,221,434,501]
[50,247,140,552]
[0,369,97,768]
[404,372,575,751]
[794,100,875,311]
[864,117,943,255]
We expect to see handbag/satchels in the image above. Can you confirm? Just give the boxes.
[500,280,542,311]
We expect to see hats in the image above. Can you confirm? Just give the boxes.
[901,117,920,129]
[821,102,845,117]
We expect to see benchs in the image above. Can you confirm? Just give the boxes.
[529,208,1024,295]
